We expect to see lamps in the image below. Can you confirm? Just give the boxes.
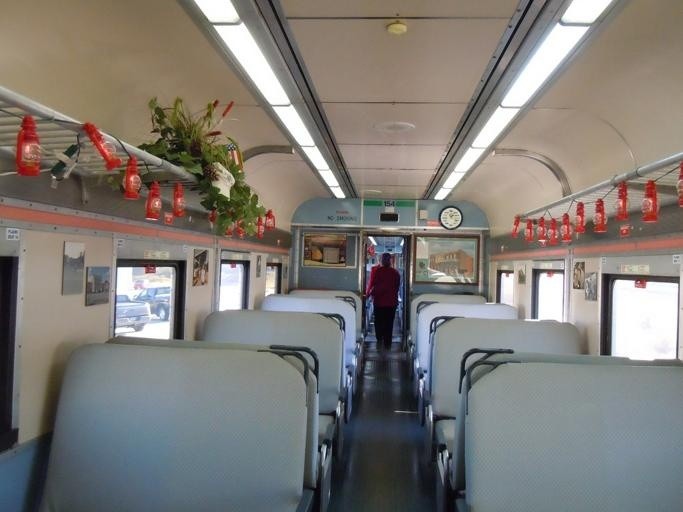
[433,0,618,199]
[195,0,346,202]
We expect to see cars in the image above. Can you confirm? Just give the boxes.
[136,286,170,319]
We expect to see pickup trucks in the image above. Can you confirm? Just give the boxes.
[115,293,151,333]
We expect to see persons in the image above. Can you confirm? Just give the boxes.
[365,253,401,353]
[366,254,381,303]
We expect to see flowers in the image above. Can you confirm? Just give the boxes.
[136,96,267,238]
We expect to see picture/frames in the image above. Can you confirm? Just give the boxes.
[440,205,463,230]
